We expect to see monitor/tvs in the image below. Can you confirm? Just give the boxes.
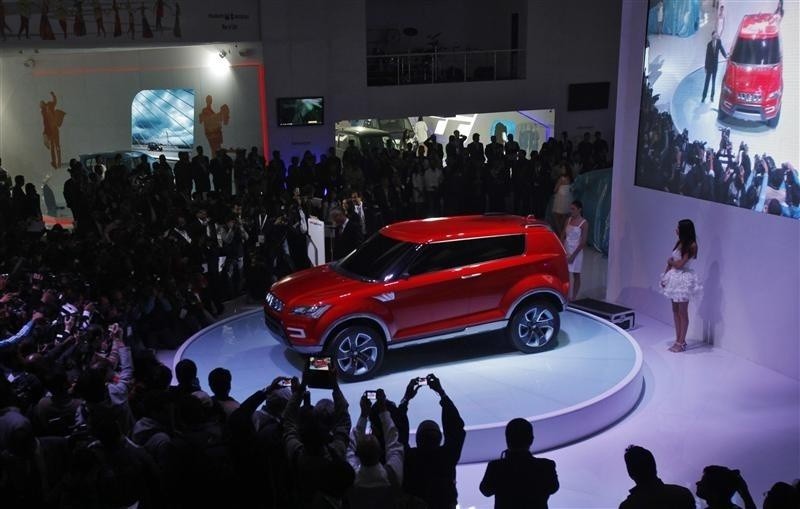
[566,82,610,110]
[276,96,325,127]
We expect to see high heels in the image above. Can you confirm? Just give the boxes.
[669,342,687,352]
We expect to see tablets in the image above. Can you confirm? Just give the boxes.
[308,354,334,371]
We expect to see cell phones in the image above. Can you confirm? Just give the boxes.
[366,390,376,399]
[418,378,429,386]
[282,378,292,387]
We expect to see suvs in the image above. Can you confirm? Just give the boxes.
[43,151,173,213]
[263,214,573,380]
[334,118,420,171]
[717,15,783,128]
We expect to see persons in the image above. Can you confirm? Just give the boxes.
[479,417,560,509]
[656,0,665,39]
[715,6,726,39]
[633,74,800,225]
[696,464,758,509]
[700,29,728,105]
[761,480,800,508]
[658,217,699,354]
[773,1,786,22]
[618,444,697,508]
